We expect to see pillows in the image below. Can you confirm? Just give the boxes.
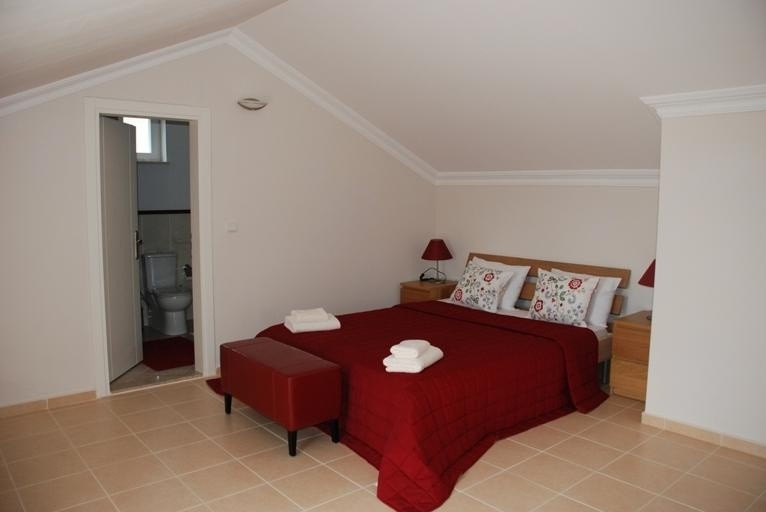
[549,267,622,329]
[528,267,600,329]
[449,258,515,314]
[471,254,532,312]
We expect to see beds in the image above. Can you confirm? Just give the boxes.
[204,251,632,512]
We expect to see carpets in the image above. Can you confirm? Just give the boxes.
[137,335,194,374]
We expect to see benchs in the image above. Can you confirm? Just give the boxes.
[217,335,342,456]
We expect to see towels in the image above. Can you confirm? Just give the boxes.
[282,313,343,334]
[389,338,431,360]
[382,346,445,374]
[290,306,329,324]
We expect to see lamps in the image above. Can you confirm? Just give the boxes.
[636,259,655,320]
[421,238,452,284]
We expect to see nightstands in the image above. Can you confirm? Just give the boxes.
[610,309,651,403]
[398,278,456,304]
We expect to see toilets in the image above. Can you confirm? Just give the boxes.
[140,250,193,335]
